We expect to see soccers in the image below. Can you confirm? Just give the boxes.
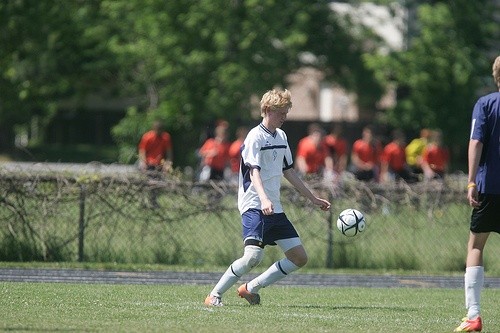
[337,208,365,237]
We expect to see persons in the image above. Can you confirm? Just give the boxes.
[453,56,500,332]
[197,119,248,208]
[294,121,450,216]
[205,89,331,307]
[138,119,173,208]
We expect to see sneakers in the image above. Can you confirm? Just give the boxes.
[454,316,482,332]
[238,283,260,305]
[205,295,224,307]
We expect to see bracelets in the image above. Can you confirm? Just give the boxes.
[467,184,477,189]
